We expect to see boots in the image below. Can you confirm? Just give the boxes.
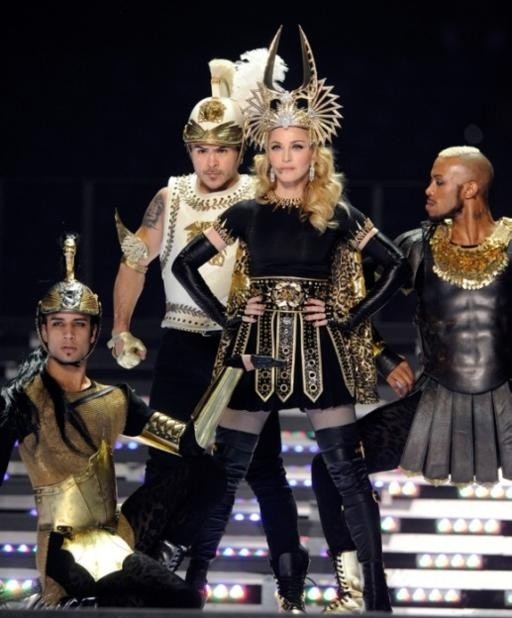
[362,555,394,616]
[269,551,308,617]
[318,549,364,616]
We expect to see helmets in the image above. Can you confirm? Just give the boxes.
[182,75,250,145]
[36,232,104,316]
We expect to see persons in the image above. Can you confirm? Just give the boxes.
[1,278,204,609]
[309,146,512,612]
[111,93,312,613]
[167,101,411,614]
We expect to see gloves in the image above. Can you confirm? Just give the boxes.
[104,328,149,372]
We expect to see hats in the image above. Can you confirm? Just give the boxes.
[245,24,343,149]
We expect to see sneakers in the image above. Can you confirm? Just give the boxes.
[157,536,190,574]
[185,556,209,609]
